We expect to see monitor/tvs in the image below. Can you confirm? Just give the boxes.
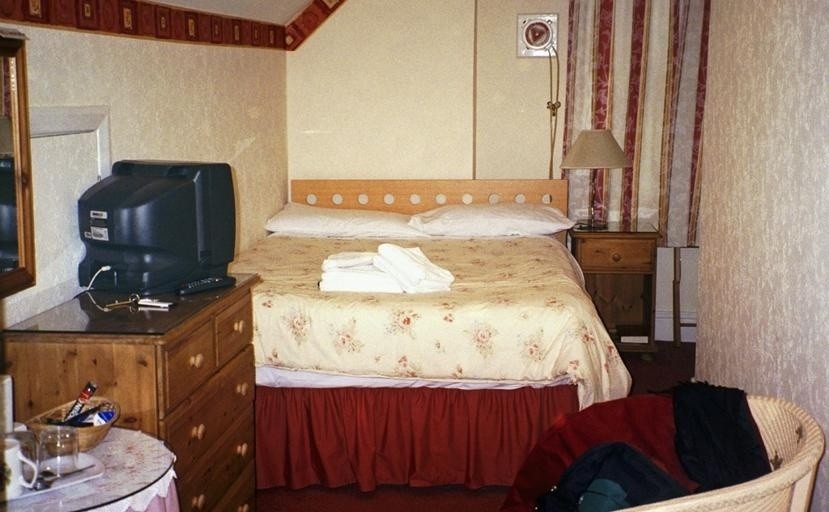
[0,157,19,271]
[78,160,236,295]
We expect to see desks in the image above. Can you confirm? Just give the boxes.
[4,421,182,511]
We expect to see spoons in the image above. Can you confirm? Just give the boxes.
[26,463,96,492]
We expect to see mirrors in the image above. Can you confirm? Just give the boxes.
[0,28,37,302]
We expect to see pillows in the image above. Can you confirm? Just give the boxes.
[410,203,578,238]
[266,201,427,241]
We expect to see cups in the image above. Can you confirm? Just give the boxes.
[0,438,37,501]
[38,426,84,479]
[5,431,37,482]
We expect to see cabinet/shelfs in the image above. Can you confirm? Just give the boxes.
[0,272,267,511]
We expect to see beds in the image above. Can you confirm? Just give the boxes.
[226,178,634,493]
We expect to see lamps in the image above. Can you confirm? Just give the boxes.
[559,128,628,230]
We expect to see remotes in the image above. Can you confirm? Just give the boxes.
[176,275,236,295]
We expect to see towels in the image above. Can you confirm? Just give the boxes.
[316,242,454,294]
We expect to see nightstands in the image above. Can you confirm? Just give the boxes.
[569,219,667,361]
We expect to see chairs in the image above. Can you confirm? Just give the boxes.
[502,386,825,512]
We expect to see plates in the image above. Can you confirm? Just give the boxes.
[0,451,105,503]
